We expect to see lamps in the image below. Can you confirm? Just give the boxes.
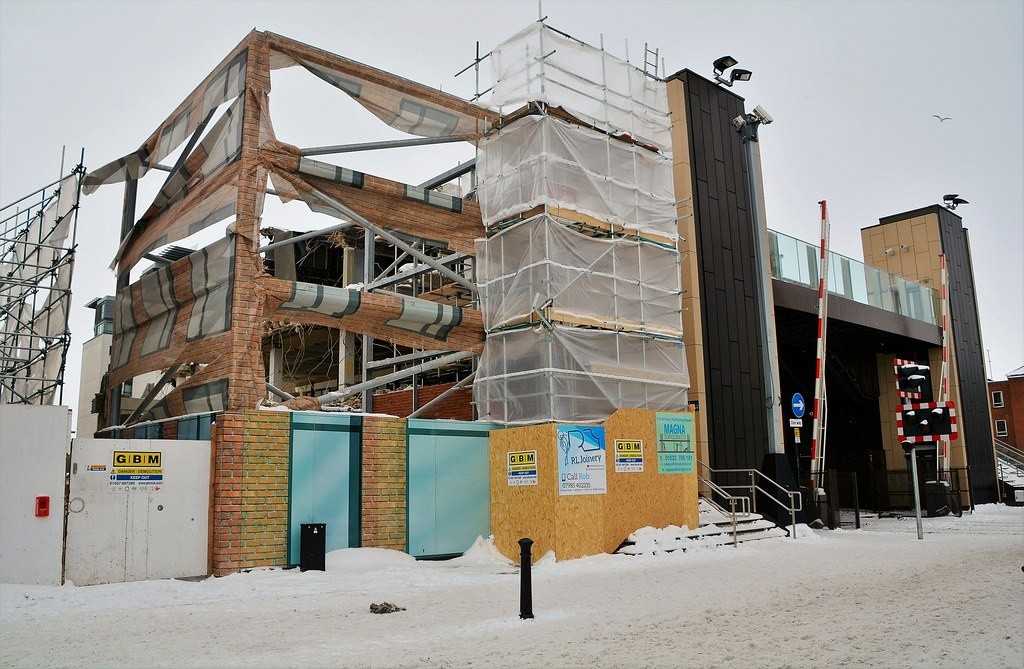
[953,199,969,204]
[713,56,738,73]
[730,69,752,81]
[943,195,959,200]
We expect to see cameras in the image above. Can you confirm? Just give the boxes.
[753,104,773,125]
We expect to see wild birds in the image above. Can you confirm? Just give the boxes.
[932,114,952,123]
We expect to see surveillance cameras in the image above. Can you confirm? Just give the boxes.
[733,115,745,130]
[900,245,909,249]
[885,248,893,255]
